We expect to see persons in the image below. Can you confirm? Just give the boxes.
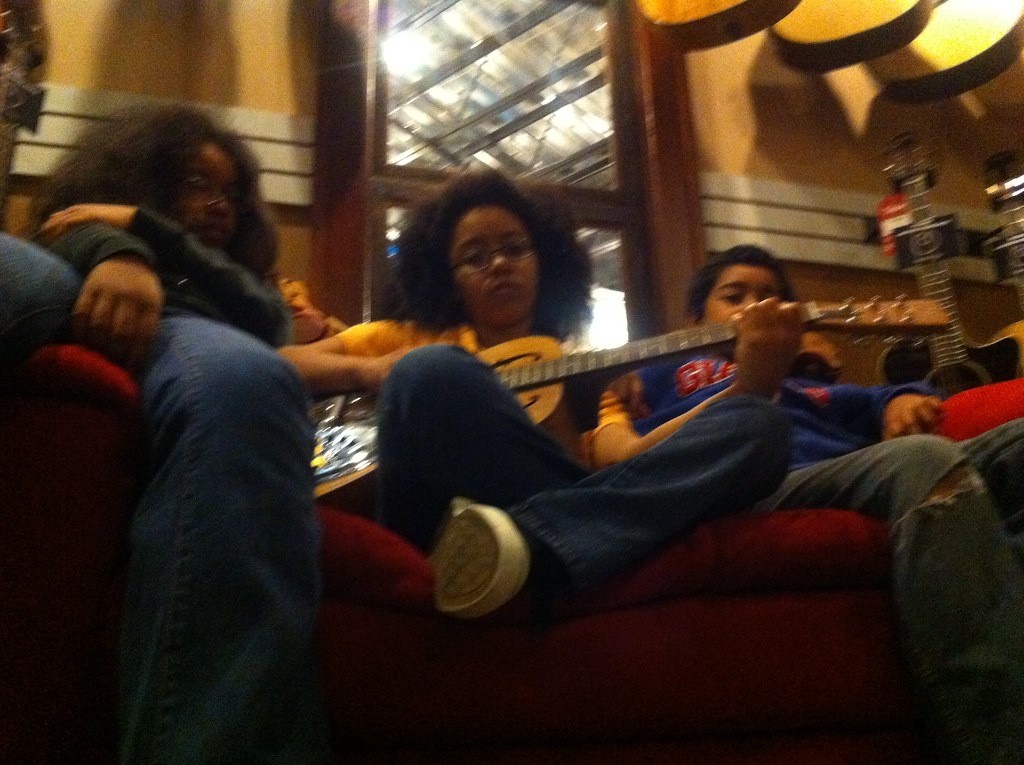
[582,245,1024,765]
[275,172,793,624]
[0,102,317,765]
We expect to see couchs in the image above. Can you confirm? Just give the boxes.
[0,326,1024,765]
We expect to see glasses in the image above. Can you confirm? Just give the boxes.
[182,173,247,212]
[444,235,538,274]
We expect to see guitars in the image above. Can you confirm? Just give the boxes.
[874,130,1023,401]
[310,294,950,496]
[977,145,1024,300]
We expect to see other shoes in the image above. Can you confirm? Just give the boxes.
[429,496,529,620]
